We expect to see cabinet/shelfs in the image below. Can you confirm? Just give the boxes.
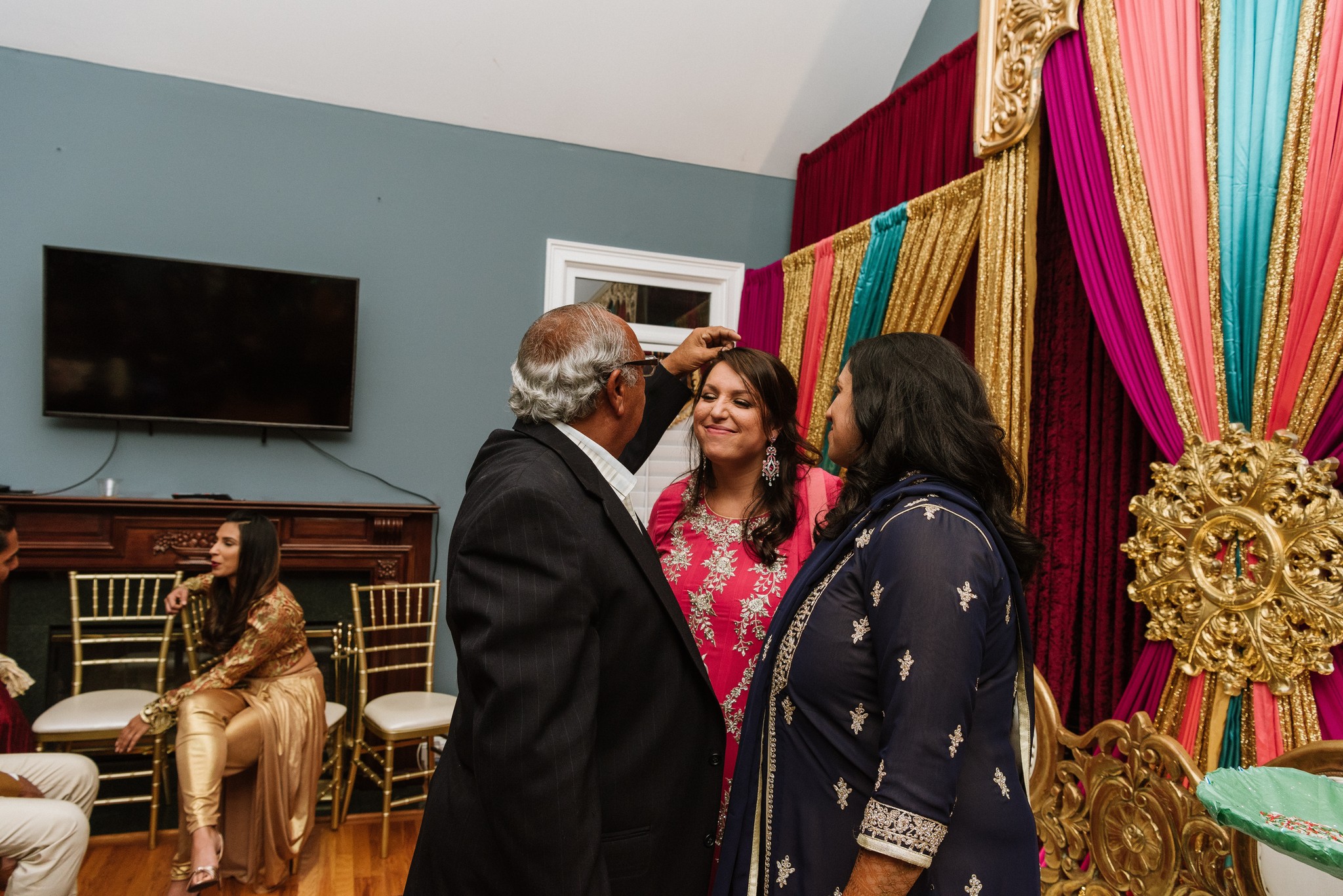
[0,491,441,787]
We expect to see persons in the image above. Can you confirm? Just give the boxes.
[713,331,1051,896]
[635,347,851,896]
[0,515,100,896]
[113,516,332,895]
[399,301,731,896]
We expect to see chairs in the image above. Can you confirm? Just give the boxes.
[176,587,349,876]
[30,569,184,851]
[337,580,463,860]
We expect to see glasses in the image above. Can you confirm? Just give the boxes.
[619,355,658,377]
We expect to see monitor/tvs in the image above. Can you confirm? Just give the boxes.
[38,243,362,435]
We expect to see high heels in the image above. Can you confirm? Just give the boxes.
[185,834,226,891]
